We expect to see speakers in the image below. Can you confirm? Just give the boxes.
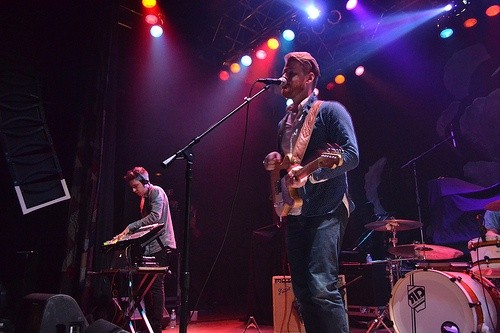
[13,293,88,333]
[84,318,130,333]
[339,212,391,307]
[272,275,348,333]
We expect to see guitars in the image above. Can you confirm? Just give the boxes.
[270,148,344,217]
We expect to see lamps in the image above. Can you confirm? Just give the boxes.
[219,0,364,89]
[434,0,500,38]
[145,10,164,37]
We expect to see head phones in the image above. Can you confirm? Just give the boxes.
[133,171,148,184]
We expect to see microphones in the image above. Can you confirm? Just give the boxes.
[450,126,457,147]
[255,77,287,87]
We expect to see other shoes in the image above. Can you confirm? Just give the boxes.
[143,326,160,333]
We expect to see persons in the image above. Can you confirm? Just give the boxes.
[114,167,176,333]
[482,203,500,239]
[260,51,361,333]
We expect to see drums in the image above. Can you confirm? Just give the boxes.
[389,268,500,333]
[469,236,500,278]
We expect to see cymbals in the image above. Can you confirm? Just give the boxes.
[364,219,423,232]
[389,244,463,260]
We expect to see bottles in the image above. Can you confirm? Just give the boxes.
[171,309,176,328]
[366,254,372,263]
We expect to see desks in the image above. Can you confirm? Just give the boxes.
[87,266,169,333]
